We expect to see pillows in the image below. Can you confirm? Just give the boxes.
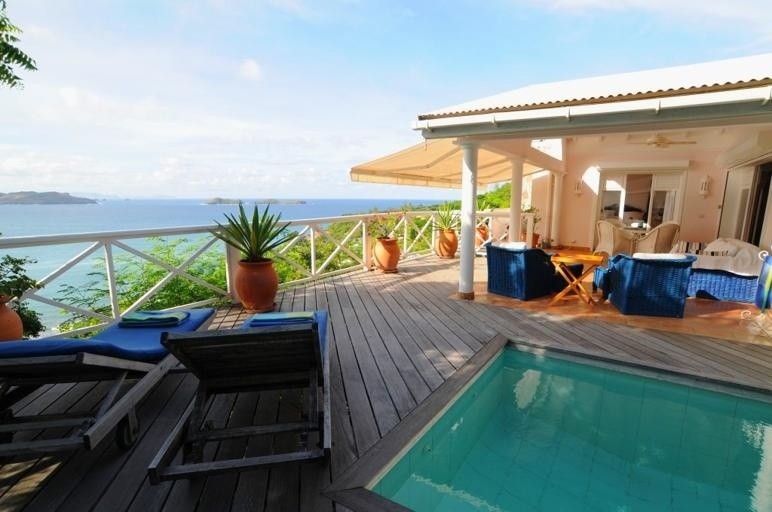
[682,237,740,257]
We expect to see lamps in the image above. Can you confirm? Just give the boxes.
[574,176,584,198]
[697,174,711,199]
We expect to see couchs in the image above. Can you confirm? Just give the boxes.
[681,237,764,303]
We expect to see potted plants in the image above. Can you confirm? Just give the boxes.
[431,203,461,259]
[521,206,550,250]
[0,254,38,341]
[475,201,495,248]
[209,200,302,314]
[371,212,401,274]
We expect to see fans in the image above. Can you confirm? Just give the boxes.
[627,132,697,151]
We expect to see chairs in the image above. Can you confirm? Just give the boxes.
[486,220,697,317]
[0,297,215,454]
[147,310,337,484]
